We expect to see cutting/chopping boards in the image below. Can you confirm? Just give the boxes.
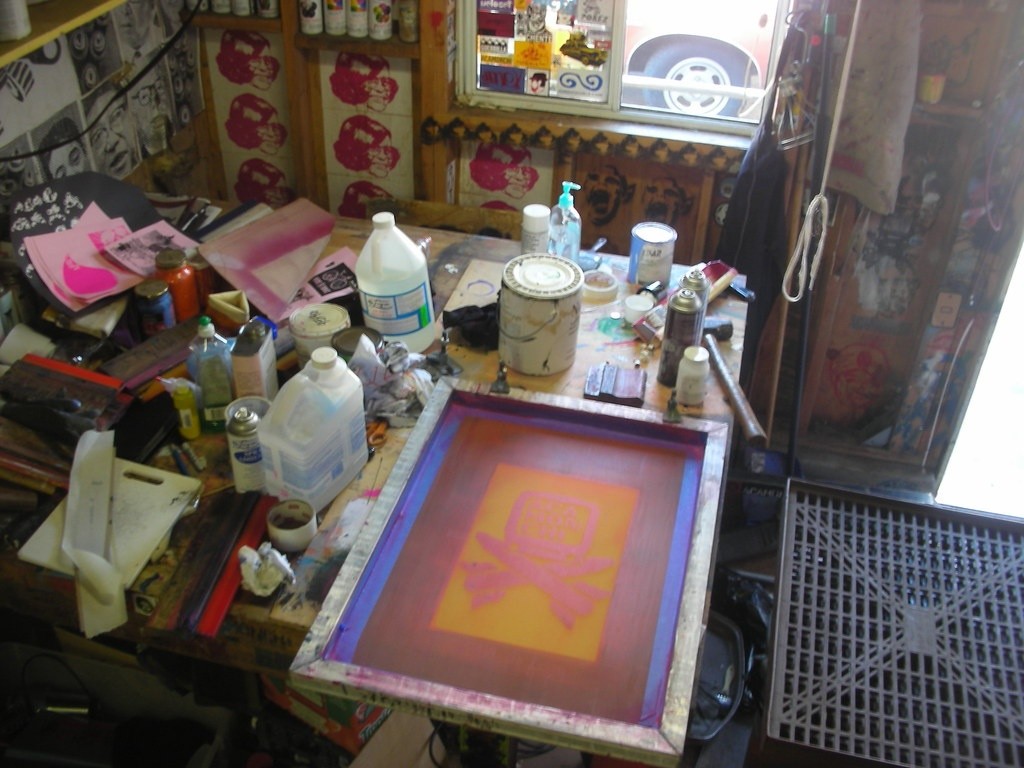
[16,458,200,588]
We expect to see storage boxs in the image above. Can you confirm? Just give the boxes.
[259,673,393,756]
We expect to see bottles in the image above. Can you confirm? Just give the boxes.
[231,318,280,403]
[185,316,238,433]
[172,377,203,440]
[256,346,370,517]
[675,344,709,406]
[288,303,385,372]
[520,203,552,258]
[187,1,419,42]
[656,269,712,388]
[353,211,436,355]
[226,406,261,494]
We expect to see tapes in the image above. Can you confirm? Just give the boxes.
[581,269,619,306]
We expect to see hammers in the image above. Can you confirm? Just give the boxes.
[701,316,768,446]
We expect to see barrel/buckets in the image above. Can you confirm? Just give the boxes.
[626,222,677,286]
[495,252,585,376]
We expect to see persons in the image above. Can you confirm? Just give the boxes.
[37,117,87,180]
[82,81,140,179]
[129,61,178,160]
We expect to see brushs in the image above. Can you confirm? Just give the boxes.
[580,237,608,270]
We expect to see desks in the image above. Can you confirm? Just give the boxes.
[2,193,750,768]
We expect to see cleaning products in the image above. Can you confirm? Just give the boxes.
[185,317,234,432]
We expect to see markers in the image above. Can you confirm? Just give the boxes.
[169,442,203,476]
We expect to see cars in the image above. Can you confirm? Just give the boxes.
[544,0,777,123]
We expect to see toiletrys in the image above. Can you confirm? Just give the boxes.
[546,181,582,264]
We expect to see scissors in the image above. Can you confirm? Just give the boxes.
[365,418,388,446]
